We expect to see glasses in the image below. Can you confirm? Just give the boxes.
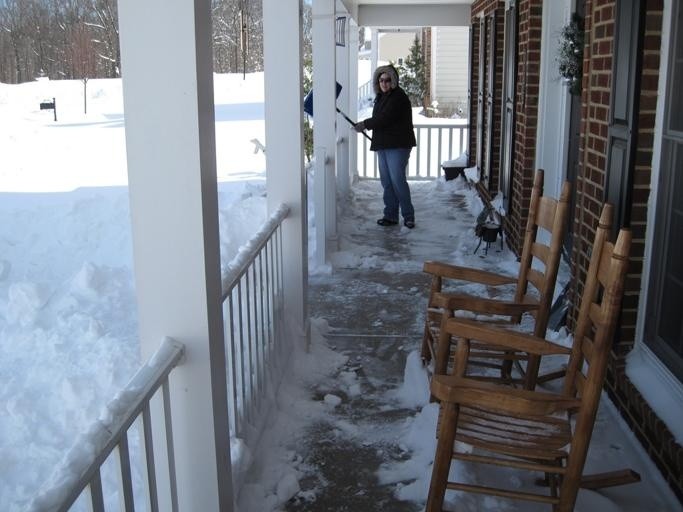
[378,77,390,82]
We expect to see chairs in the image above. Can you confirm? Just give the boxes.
[421,202,642,512]
[421,168,574,407]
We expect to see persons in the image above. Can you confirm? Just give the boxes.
[350,64,414,229]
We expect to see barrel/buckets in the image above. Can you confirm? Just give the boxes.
[481,222,499,242]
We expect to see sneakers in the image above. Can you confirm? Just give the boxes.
[405,221,414,228]
[377,218,398,226]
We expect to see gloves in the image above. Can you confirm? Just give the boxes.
[354,121,366,132]
